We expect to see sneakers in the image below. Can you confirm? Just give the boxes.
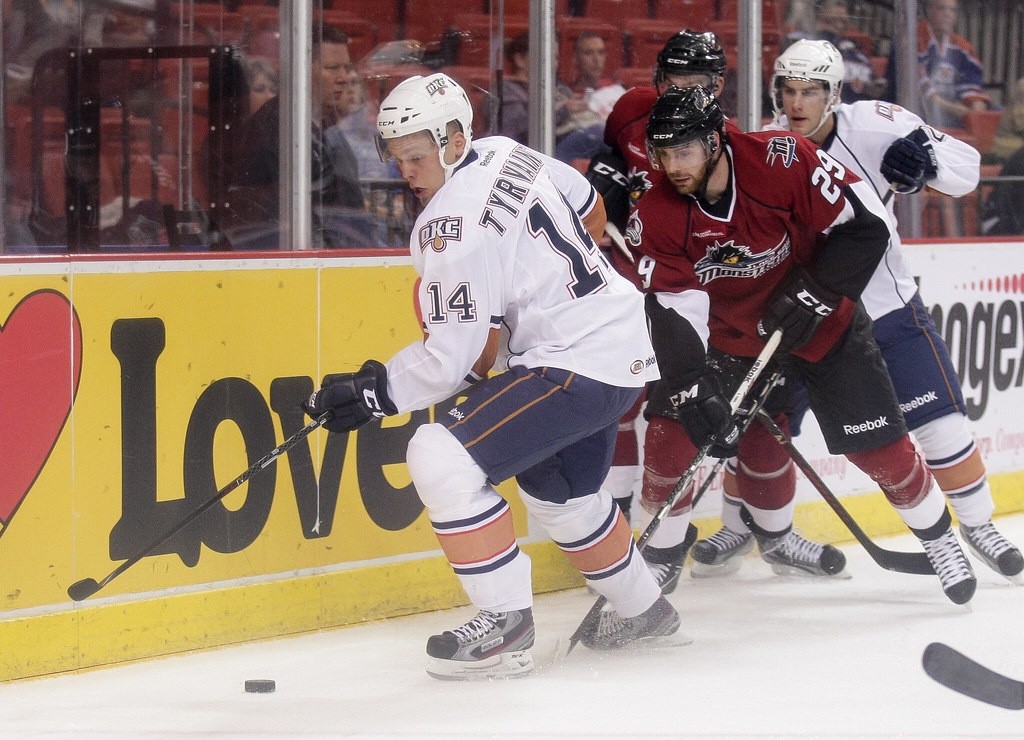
[580,591,693,651]
[740,505,852,578]
[959,520,1024,586]
[918,524,976,612]
[425,609,535,680]
[588,522,698,596]
[690,525,755,578]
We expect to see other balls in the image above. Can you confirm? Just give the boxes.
[245,679,275,693]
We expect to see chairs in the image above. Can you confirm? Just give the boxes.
[0,0,1024,251]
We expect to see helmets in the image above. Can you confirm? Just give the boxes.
[768,38,845,115]
[657,29,728,87]
[644,84,726,171]
[376,72,473,168]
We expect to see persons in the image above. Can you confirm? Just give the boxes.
[482,24,594,147]
[879,0,1003,128]
[570,33,627,127]
[244,20,369,250]
[326,27,483,248]
[323,64,362,129]
[198,56,277,191]
[300,30,1024,662]
[780,0,873,105]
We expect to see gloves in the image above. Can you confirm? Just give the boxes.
[300,359,398,433]
[664,367,745,458]
[880,128,938,194]
[756,265,842,354]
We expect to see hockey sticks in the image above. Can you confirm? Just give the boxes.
[692,182,900,510]
[758,409,937,577]
[921,639,1023,711]
[65,411,328,603]
[551,330,785,671]
